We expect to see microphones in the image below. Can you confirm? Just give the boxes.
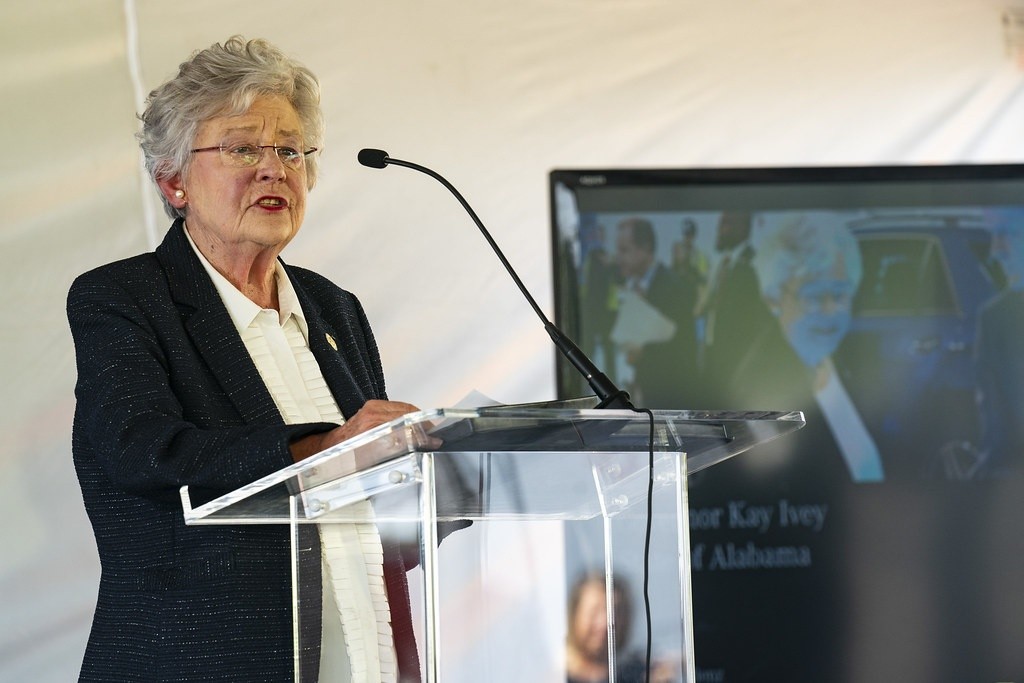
[358,148,634,412]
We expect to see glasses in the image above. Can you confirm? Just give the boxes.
[191,141,318,170]
[771,281,848,312]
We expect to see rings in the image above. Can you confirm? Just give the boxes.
[387,437,403,448]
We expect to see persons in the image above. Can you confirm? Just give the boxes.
[67,37,480,683]
[568,215,907,683]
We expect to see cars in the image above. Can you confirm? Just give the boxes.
[831,209,1024,468]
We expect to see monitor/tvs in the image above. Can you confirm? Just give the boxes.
[549,161,1024,683]
